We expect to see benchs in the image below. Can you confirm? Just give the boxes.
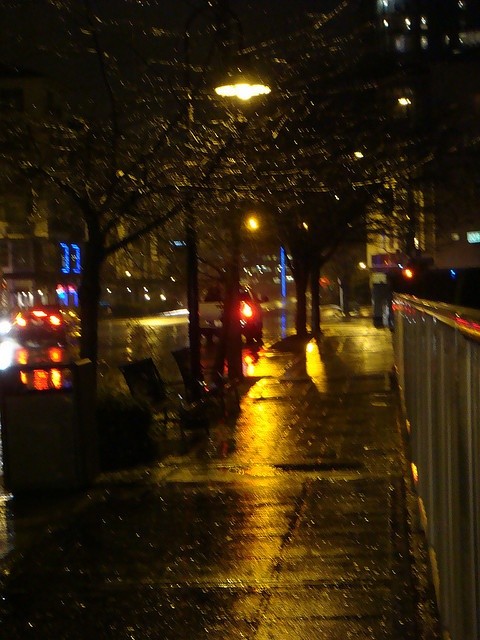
[117,356,211,437]
[171,348,228,419]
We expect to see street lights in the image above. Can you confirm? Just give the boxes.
[183,71,271,400]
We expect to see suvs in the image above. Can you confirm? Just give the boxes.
[196,284,269,341]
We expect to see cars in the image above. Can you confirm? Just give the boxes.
[11,306,69,349]
